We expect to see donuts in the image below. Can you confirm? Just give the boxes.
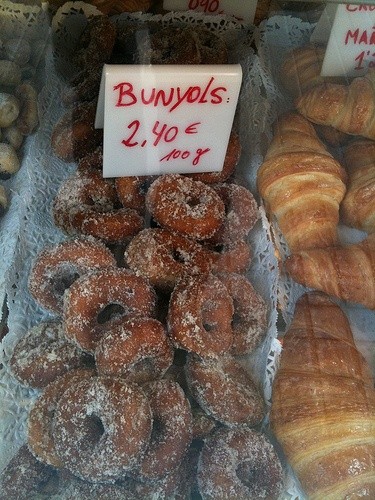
[1,0,282,500]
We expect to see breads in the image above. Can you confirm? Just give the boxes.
[258,41,375,500]
